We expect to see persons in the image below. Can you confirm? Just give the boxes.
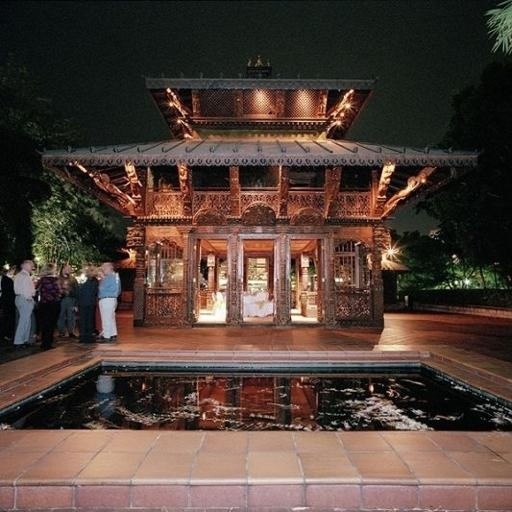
[0,259,122,352]
[95,373,116,418]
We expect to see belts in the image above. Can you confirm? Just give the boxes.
[98,296,117,301]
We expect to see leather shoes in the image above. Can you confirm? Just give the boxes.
[15,332,118,351]
